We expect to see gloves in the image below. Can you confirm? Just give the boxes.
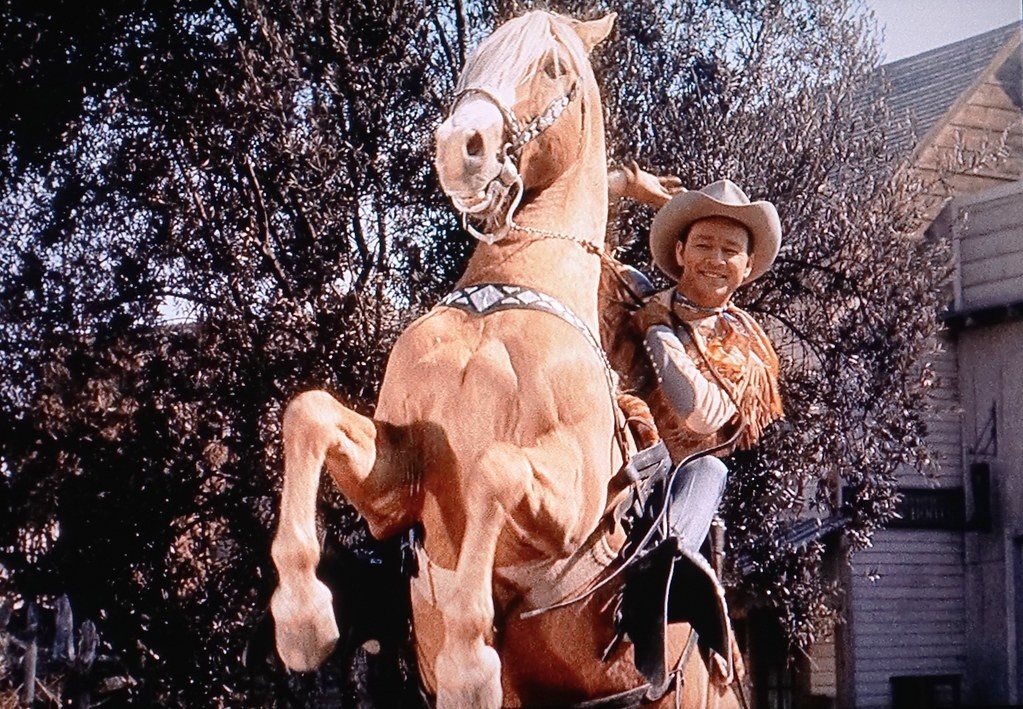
[637,300,671,331]
[614,160,686,205]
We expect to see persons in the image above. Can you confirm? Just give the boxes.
[597,158,783,566]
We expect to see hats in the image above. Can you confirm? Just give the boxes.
[649,180,783,287]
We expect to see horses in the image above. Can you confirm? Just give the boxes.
[268,9,760,708]
